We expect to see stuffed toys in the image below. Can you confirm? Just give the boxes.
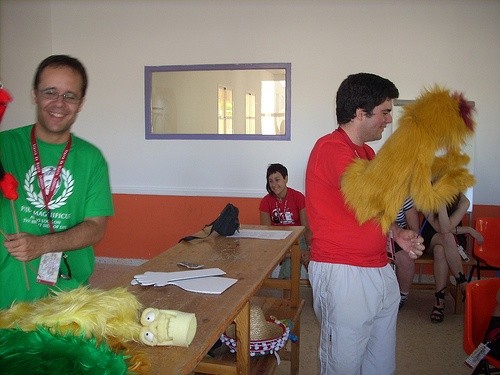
[0,79,20,203]
[336,83,479,236]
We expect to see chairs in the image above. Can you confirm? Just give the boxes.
[463,278,500,370]
[473,217,500,279]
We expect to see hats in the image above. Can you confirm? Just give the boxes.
[223,303,286,342]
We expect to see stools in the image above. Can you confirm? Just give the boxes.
[415,255,477,291]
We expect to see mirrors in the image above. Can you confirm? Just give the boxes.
[144,62,292,141]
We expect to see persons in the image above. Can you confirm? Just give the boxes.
[468,285,500,374]
[390,193,421,310]
[420,191,484,324]
[0,54,114,310]
[305,72,425,375]
[258,164,312,299]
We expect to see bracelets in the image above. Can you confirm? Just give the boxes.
[456,226,458,235]
[388,224,397,240]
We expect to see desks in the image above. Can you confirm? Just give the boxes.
[62,224,306,375]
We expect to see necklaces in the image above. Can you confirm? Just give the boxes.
[274,191,285,203]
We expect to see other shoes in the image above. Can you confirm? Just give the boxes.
[399,292,410,310]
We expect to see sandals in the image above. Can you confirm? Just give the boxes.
[454,272,469,302]
[430,287,447,322]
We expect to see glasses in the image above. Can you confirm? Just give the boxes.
[36,88,82,103]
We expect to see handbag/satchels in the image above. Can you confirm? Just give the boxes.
[178,202,240,243]
